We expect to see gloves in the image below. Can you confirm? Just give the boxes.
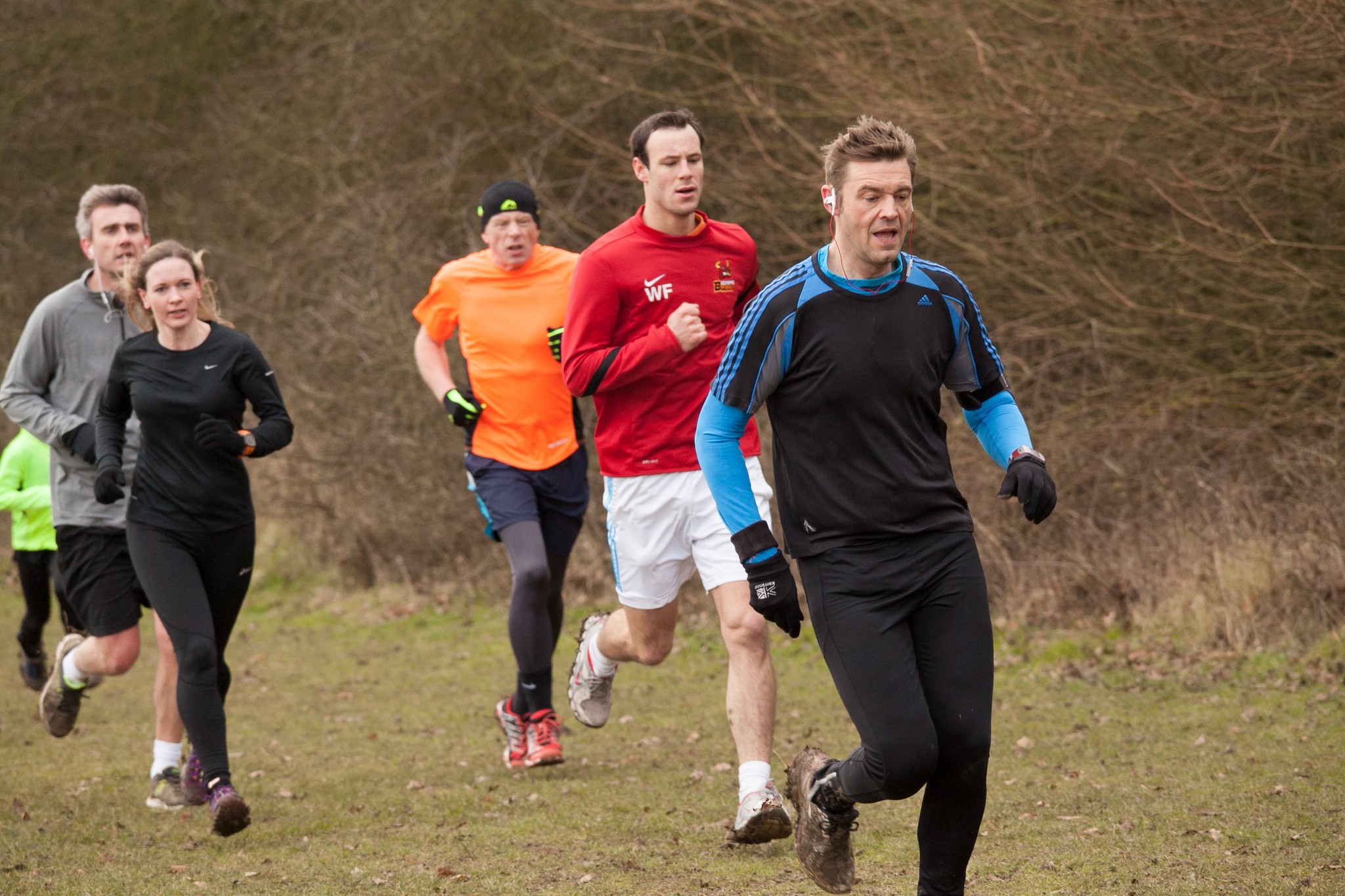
[443,387,487,429]
[741,549,804,639]
[997,458,1058,526]
[65,422,96,465]
[547,326,564,363]
[94,469,126,505]
[194,413,245,457]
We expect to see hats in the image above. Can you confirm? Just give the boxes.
[478,180,540,232]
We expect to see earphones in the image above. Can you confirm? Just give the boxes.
[909,195,914,213]
[143,242,148,252]
[824,186,839,215]
[88,245,96,258]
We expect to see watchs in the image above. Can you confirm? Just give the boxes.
[235,430,256,458]
[1008,444,1046,467]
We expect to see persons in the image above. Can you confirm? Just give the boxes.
[0,181,191,816]
[693,115,1060,896]
[0,424,90,693]
[407,180,591,771]
[557,109,796,848]
[85,236,295,839]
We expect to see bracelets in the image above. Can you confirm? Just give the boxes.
[731,520,779,565]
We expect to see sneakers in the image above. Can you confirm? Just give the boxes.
[787,746,859,894]
[180,754,210,806]
[145,767,188,811]
[567,609,618,729]
[38,633,92,739]
[524,706,566,766]
[733,777,794,844]
[205,776,251,837]
[495,696,529,769]
[19,642,47,689]
